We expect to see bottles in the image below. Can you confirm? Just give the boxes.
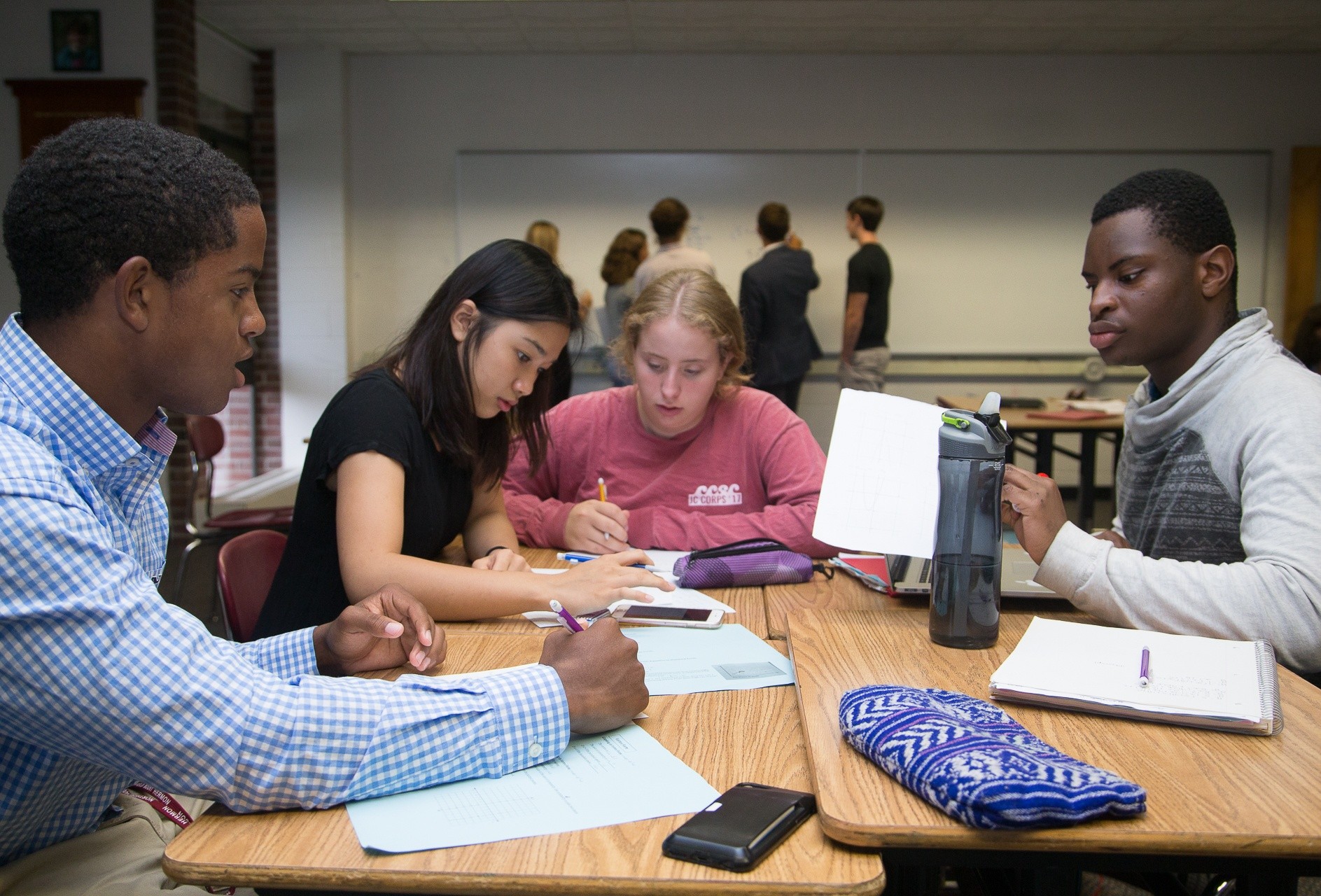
[927,389,1013,651]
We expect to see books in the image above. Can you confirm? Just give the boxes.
[951,619,1284,736]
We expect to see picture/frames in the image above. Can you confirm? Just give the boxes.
[47,7,104,73]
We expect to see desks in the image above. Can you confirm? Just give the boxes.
[937,393,1125,535]
[162,546,1321,896]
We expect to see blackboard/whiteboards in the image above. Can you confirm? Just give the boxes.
[454,146,1275,359]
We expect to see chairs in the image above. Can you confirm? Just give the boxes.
[213,530,289,643]
[171,413,298,609]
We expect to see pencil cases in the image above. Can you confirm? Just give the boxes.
[672,538,834,589]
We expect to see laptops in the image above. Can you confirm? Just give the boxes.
[884,547,1071,600]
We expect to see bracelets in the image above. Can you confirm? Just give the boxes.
[485,546,509,557]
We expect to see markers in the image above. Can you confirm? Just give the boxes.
[1013,473,1048,513]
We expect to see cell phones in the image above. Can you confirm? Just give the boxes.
[663,782,818,874]
[612,604,725,629]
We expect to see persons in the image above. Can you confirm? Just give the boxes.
[513,219,593,413]
[837,193,894,396]
[248,238,675,675]
[953,168,1321,896]
[498,269,861,559]
[739,201,825,415]
[600,197,716,387]
[0,117,650,896]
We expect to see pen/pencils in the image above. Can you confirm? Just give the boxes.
[556,553,662,573]
[598,477,608,539]
[1140,644,1150,687]
[550,599,585,634]
